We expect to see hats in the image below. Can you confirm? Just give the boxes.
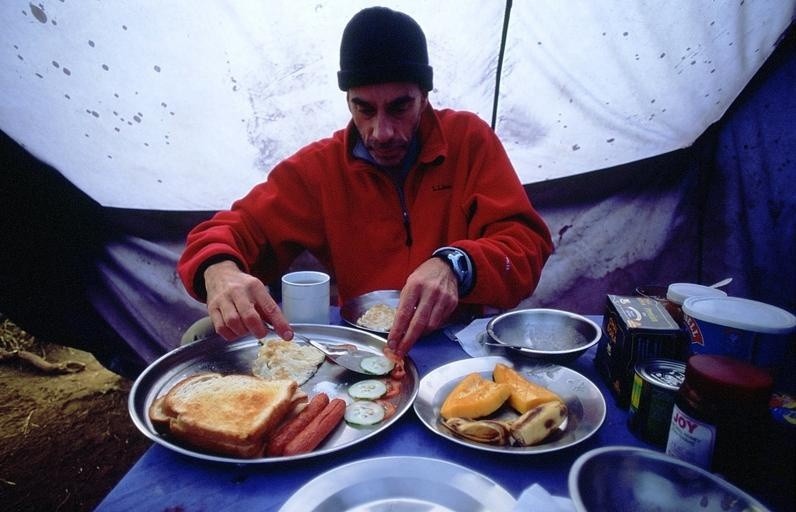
[338,6,434,90]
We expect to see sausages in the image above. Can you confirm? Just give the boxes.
[265,393,329,456]
[282,398,346,456]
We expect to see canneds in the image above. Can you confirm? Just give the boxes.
[625,358,687,451]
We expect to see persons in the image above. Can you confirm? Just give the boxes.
[177,6,555,357]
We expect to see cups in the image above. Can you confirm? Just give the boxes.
[280,272,331,325]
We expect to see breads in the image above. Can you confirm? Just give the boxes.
[148,372,308,459]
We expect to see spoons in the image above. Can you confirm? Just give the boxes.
[263,322,389,378]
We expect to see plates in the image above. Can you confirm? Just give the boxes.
[127,320,421,465]
[276,454,521,511]
[414,356,609,455]
[338,288,428,333]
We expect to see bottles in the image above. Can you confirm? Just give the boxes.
[666,352,774,490]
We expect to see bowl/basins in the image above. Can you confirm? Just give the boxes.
[487,308,604,359]
[568,446,772,510]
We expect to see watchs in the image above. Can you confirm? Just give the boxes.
[431,249,472,289]
